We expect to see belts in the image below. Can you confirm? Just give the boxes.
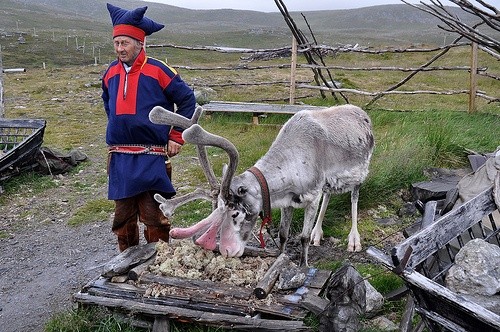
[107,146,166,157]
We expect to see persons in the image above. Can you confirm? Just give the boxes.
[101,2,196,253]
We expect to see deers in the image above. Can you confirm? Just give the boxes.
[148,104,377,258]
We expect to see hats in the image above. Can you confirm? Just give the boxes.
[106,2,164,43]
